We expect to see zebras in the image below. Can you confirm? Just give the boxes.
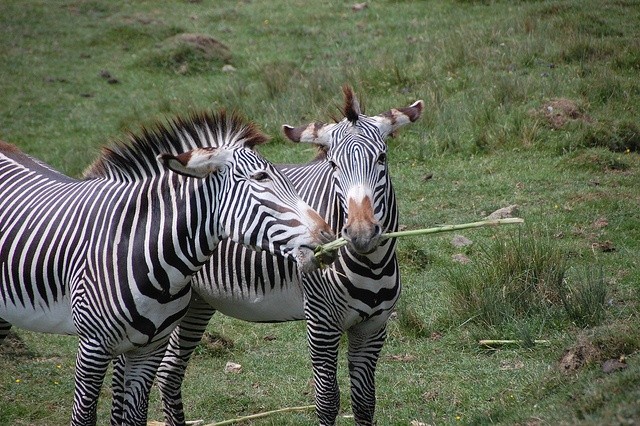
[108,81,426,425]
[0,100,341,425]
